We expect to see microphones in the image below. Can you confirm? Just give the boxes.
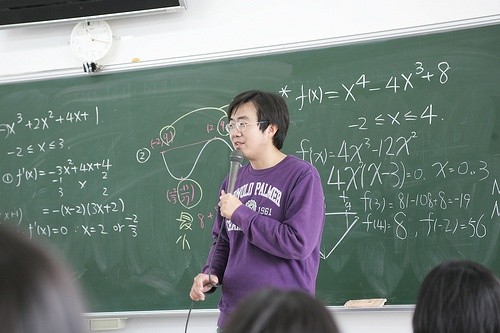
[226,150,243,196]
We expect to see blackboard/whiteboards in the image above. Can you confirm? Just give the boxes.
[0,12,500,317]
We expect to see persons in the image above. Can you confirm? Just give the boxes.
[412,260,500,333]
[0,223,89,333]
[189,90,326,333]
[218,287,341,333]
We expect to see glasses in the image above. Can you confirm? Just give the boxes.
[226,121,265,132]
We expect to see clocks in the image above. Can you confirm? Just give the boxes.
[70,19,114,61]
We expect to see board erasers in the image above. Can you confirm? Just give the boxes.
[342,299,388,310]
